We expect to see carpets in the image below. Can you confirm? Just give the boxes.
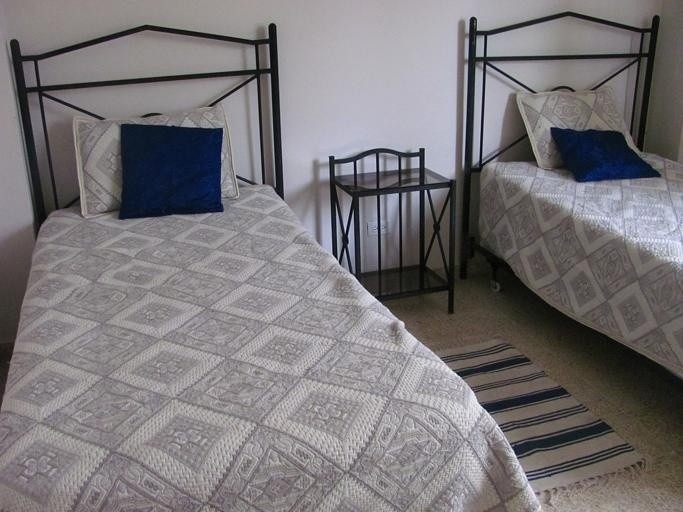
[433,335,650,505]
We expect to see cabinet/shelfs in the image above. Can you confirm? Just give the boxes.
[327,149,457,315]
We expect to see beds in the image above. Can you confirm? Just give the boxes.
[0,23,484,512]
[458,10,683,384]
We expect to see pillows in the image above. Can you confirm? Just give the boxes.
[118,123,224,220]
[71,103,239,218]
[550,127,661,182]
[516,86,643,169]
